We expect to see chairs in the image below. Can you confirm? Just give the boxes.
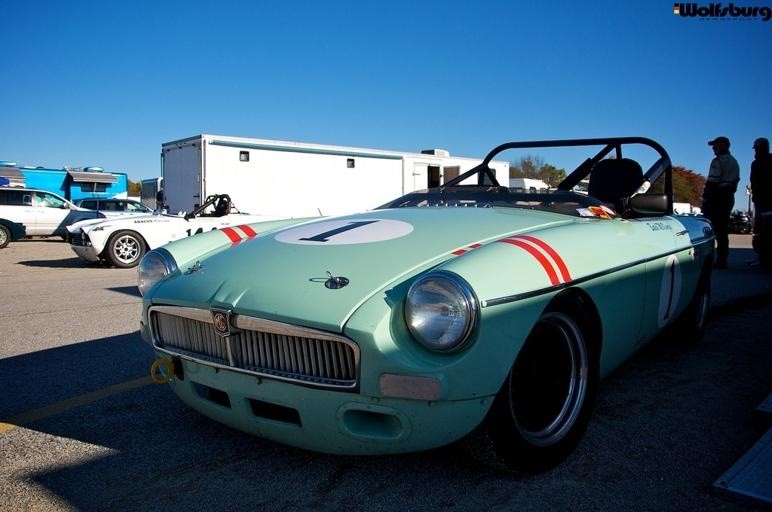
[211,199,229,216]
[586,158,643,214]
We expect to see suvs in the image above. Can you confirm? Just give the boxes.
[0,186,99,250]
[77,198,160,223]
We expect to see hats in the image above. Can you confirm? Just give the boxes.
[751,138,769,149]
[707,136,732,146]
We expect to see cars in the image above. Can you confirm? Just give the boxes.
[680,205,760,241]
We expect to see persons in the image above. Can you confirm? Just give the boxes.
[700,136,740,270]
[743,137,772,269]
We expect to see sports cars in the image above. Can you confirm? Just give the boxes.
[138,135,717,455]
[62,191,268,271]
